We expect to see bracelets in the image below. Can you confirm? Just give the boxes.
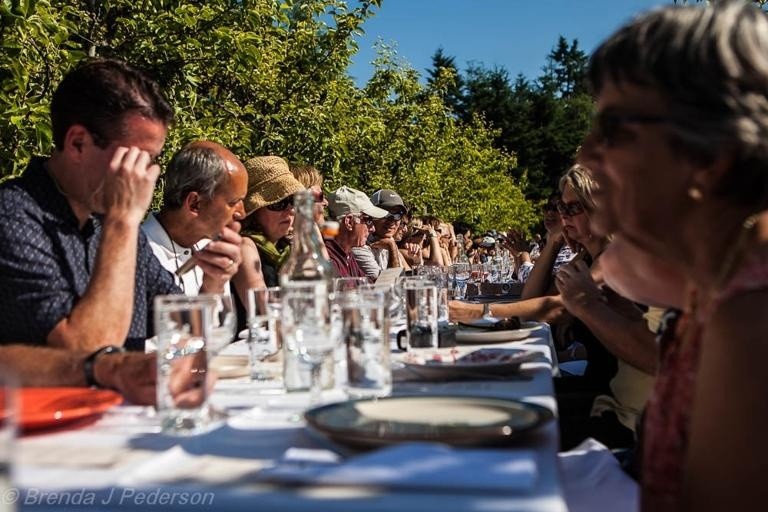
[83,343,125,390]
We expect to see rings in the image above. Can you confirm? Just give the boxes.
[223,258,236,273]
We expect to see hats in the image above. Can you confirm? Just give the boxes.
[370,189,408,215]
[240,154,306,218]
[328,185,389,219]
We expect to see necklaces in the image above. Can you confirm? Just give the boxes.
[674,211,758,365]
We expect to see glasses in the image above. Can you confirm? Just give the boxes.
[435,228,442,234]
[383,211,404,222]
[541,203,559,214]
[554,198,590,217]
[266,195,295,212]
[313,191,326,204]
[584,108,725,150]
[457,315,522,331]
[359,216,374,226]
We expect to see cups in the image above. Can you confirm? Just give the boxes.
[153,294,234,435]
[302,396,555,455]
[247,277,437,396]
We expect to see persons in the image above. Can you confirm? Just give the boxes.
[122,137,669,465]
[2,58,245,356]
[1,336,218,411]
[573,0,768,509]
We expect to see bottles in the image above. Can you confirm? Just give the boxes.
[437,233,513,323]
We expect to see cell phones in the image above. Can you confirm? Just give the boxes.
[175,238,210,277]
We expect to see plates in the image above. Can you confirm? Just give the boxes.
[0,386,123,430]
[446,319,542,343]
[397,344,546,381]
